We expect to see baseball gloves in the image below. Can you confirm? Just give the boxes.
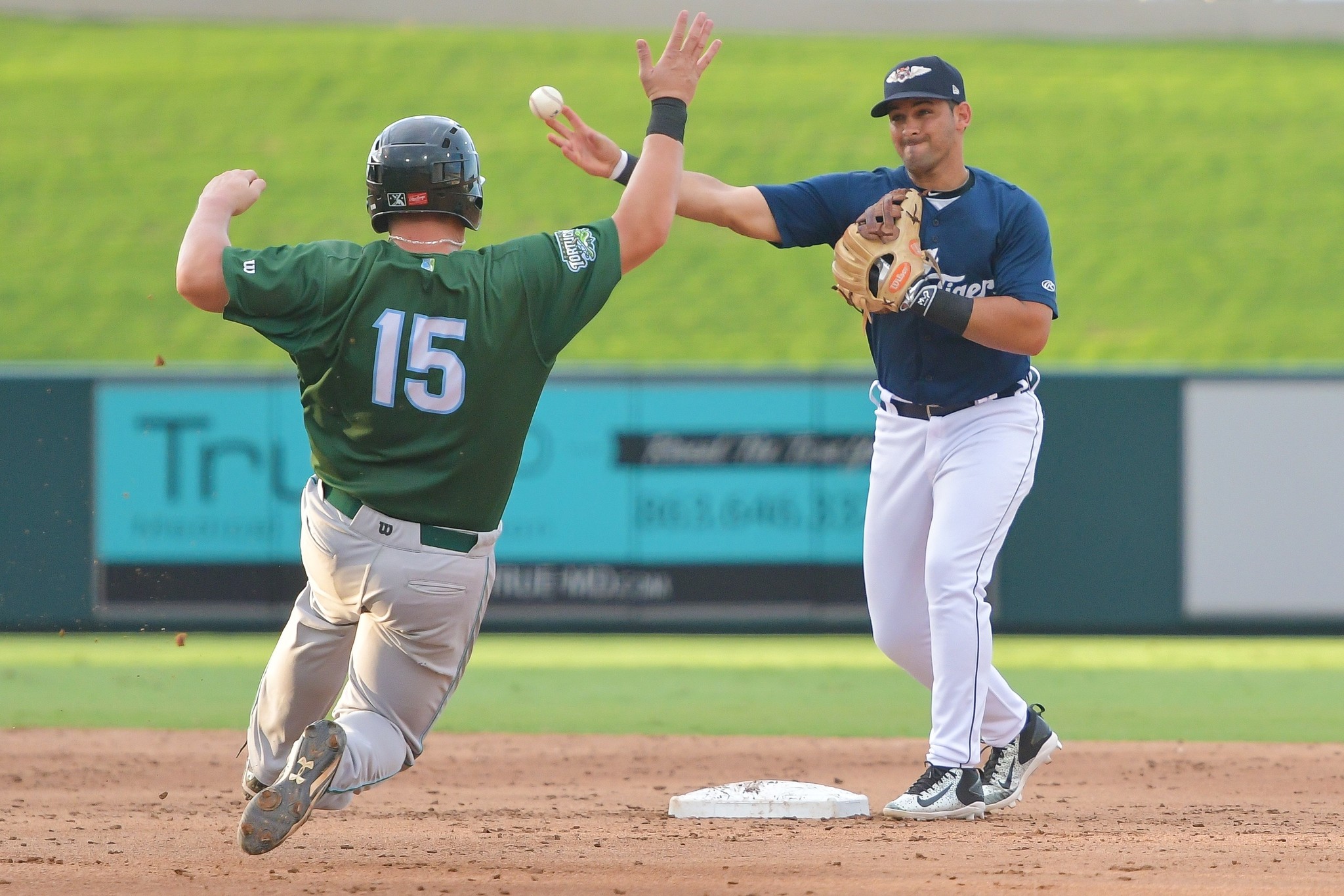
[833,187,927,313]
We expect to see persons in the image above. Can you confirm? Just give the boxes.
[545,56,1062,821]
[174,10,720,855]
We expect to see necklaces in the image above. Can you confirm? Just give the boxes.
[388,235,467,247]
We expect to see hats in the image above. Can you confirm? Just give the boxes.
[871,57,966,118]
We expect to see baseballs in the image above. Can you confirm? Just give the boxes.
[529,86,563,119]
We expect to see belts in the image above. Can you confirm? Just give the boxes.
[879,375,1031,421]
[323,485,478,552]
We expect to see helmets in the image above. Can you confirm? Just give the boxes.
[366,115,486,234]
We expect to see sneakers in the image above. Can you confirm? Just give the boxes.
[979,704,1062,815]
[241,719,348,855]
[883,760,986,821]
[243,759,268,800]
